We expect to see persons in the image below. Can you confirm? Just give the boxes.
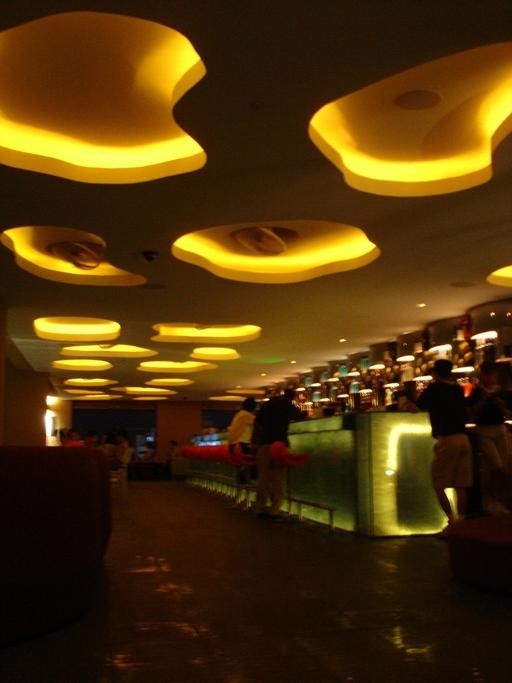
[323,387,420,418]
[416,359,512,528]
[199,425,230,436]
[167,440,179,463]
[59,428,133,471]
[144,440,156,462]
[227,387,312,522]
